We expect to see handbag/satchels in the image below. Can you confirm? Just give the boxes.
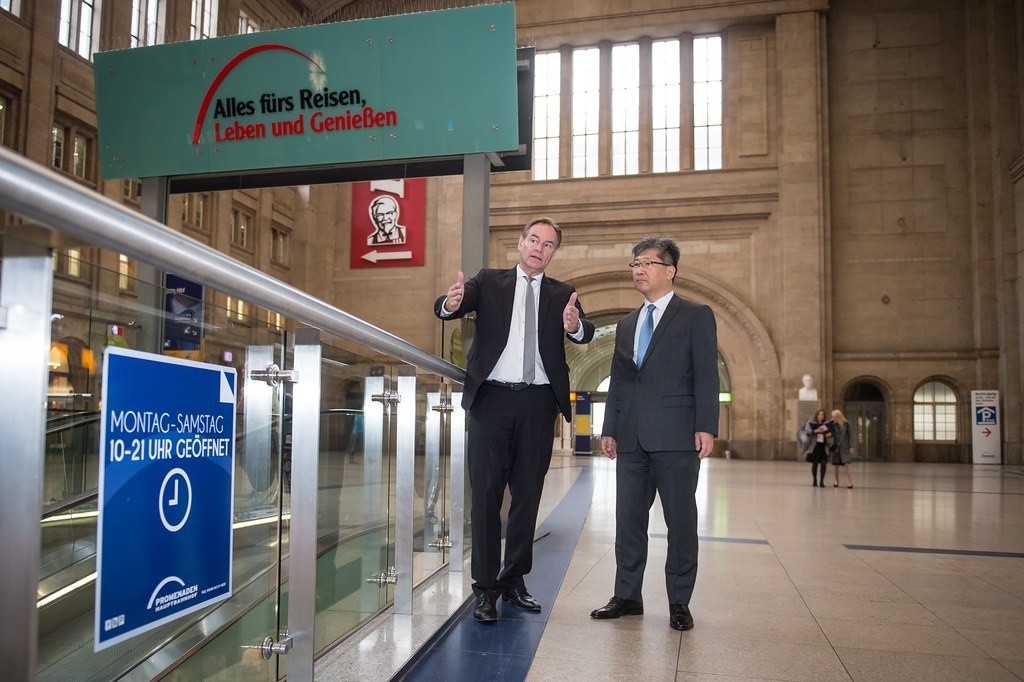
[797,419,812,450]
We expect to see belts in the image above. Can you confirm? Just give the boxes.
[486,379,549,390]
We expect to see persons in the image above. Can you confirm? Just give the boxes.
[802,408,831,487]
[798,375,818,401]
[349,406,364,463]
[434,217,595,622]
[825,409,855,489]
[589,237,720,630]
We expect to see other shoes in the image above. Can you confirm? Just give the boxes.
[813,478,818,487]
[819,482,825,487]
[834,482,839,487]
[847,484,853,488]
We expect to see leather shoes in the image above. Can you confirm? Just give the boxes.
[472,592,497,624]
[669,602,694,631]
[502,583,541,612]
[590,596,644,619]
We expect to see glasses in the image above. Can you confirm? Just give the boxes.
[628,260,671,268]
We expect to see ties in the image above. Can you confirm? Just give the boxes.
[523,276,537,385]
[636,304,657,368]
